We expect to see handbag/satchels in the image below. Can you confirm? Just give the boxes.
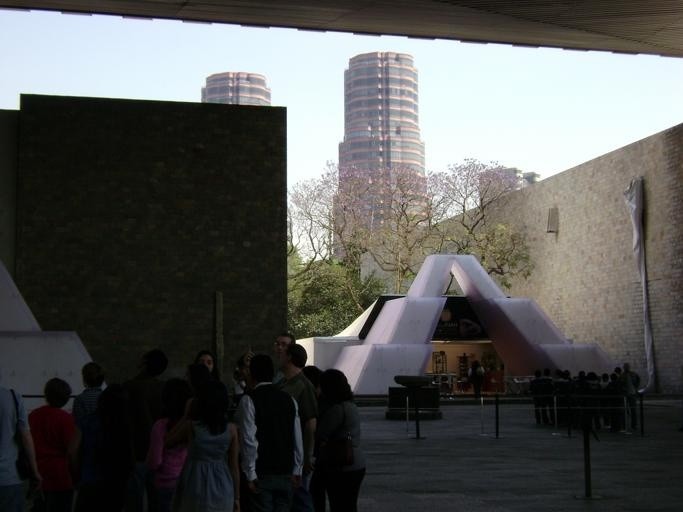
[17,449,32,482]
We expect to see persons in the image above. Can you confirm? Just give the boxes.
[1,334,366,512]
[530,363,642,434]
[468,360,505,399]
